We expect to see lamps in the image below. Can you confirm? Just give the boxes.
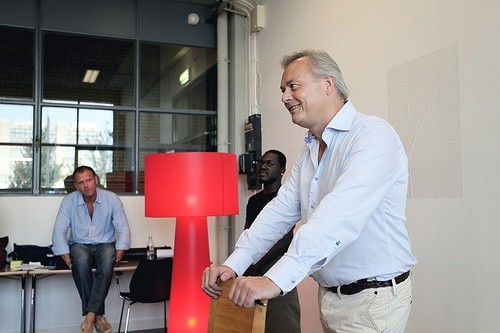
[145,152,239,333]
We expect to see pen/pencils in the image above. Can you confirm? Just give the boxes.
[254,299,267,307]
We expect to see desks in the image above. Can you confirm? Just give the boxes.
[29,261,214,333]
[0,270,28,333]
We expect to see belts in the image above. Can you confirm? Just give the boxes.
[326,270,410,295]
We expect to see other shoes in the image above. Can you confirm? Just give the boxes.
[80,321,94,333]
[95,316,112,333]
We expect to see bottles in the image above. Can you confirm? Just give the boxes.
[147,236,154,260]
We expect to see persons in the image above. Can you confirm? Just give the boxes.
[51,166,132,333]
[242,150,301,333]
[64,175,77,193]
[200,48,418,333]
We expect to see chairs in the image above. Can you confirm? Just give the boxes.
[118,257,172,333]
[208,277,268,333]
